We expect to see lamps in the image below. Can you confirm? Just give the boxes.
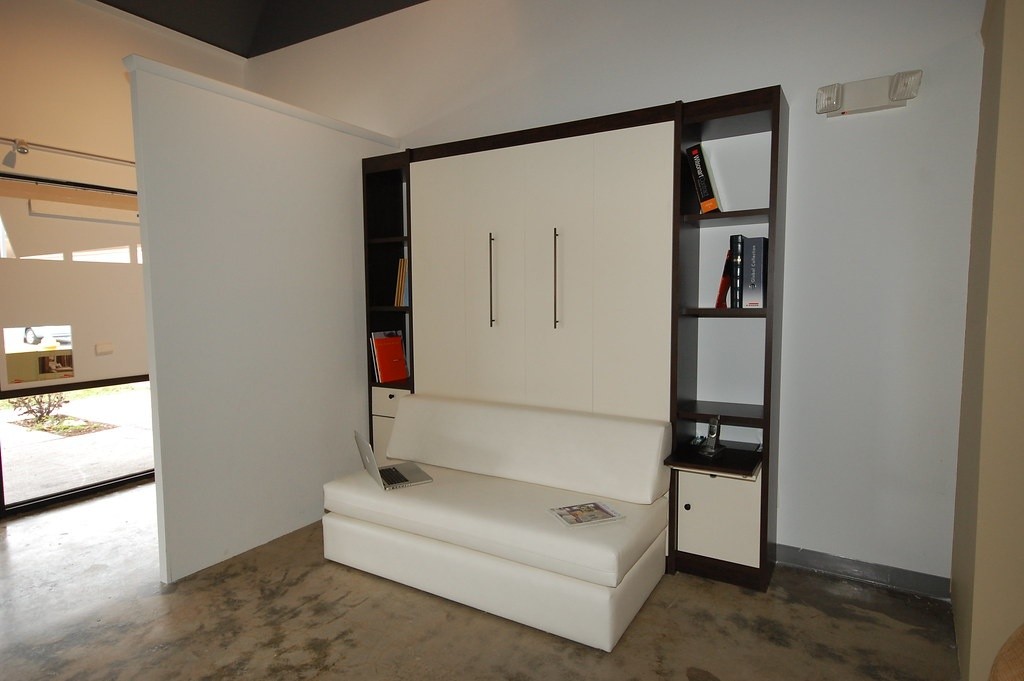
[815,68,922,118]
[15,137,29,154]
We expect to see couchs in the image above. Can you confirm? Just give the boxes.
[322,392,671,652]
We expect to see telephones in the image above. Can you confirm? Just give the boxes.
[699,414,725,457]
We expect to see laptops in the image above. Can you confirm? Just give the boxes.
[354,433,433,490]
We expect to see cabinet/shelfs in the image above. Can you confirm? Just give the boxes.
[405,100,684,421]
[362,148,414,470]
[665,85,788,594]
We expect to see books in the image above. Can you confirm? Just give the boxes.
[369,329,409,384]
[394,258,409,306]
[546,500,624,528]
